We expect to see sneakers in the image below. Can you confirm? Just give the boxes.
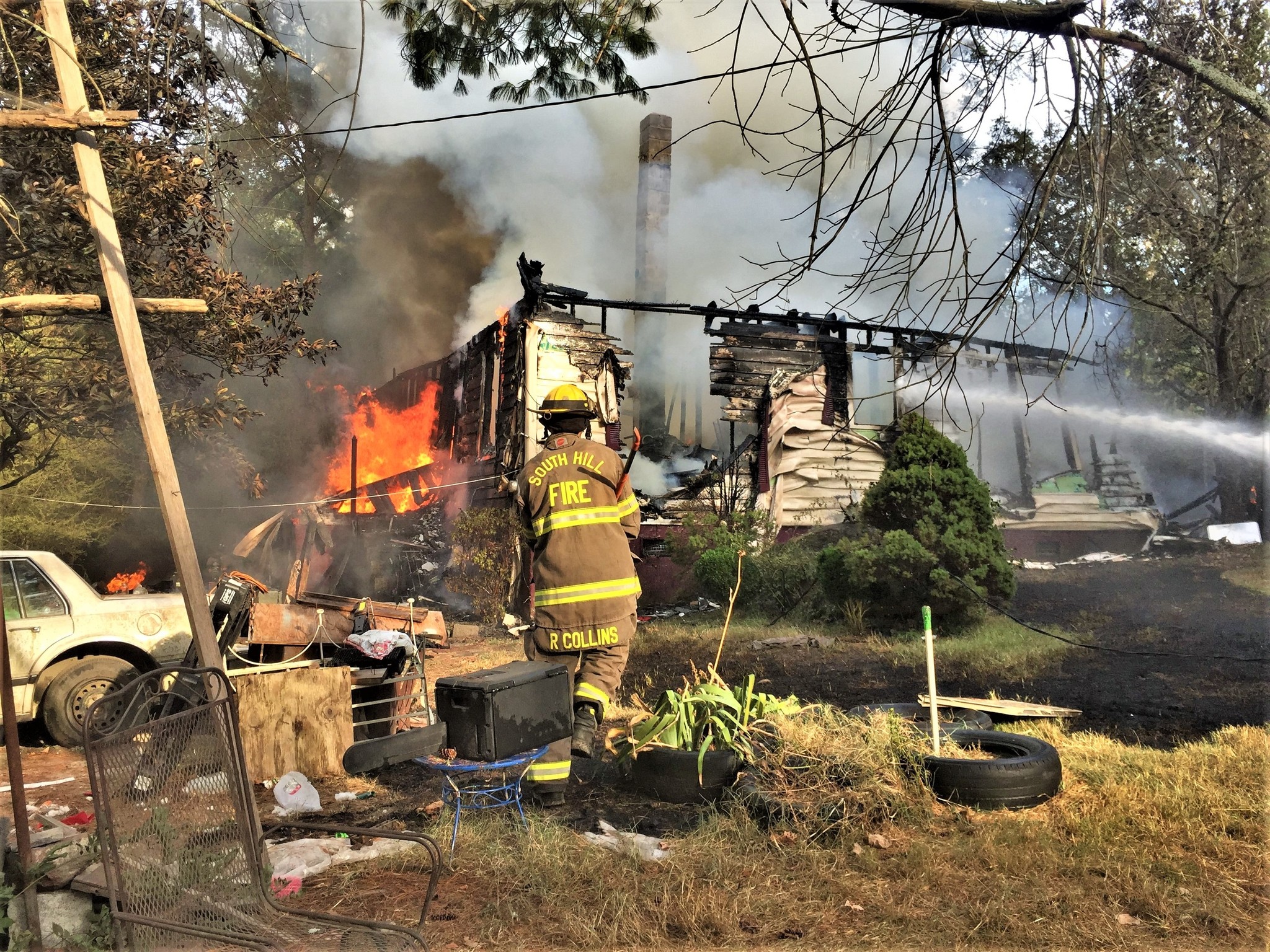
[530,789,566,813]
[572,704,598,760]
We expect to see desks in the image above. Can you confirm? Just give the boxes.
[411,744,548,856]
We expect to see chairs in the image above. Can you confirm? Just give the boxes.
[84,666,441,952]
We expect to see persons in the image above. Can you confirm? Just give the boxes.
[517,384,642,806]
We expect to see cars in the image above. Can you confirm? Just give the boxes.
[1,550,229,746]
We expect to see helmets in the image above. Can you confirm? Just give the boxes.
[526,383,600,420]
